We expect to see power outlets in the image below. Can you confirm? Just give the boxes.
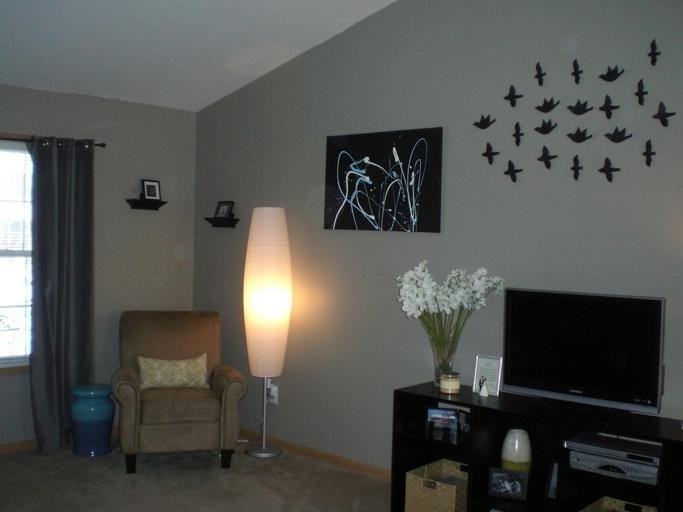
[267,385,278,405]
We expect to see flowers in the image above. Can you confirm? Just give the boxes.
[396,260,504,382]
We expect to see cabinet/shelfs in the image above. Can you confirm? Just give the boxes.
[393,390,682,512]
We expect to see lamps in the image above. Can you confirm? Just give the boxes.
[244,207,292,459]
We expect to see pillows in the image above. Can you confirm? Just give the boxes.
[136,354,210,391]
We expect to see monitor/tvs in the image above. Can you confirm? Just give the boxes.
[500,288,665,416]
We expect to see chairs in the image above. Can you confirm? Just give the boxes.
[112,311,248,473]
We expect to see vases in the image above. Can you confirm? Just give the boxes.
[71,385,115,456]
[430,336,458,387]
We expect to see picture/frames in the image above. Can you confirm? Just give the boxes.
[215,201,234,217]
[489,468,529,500]
[473,354,503,397]
[142,179,161,199]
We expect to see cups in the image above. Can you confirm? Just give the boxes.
[501,429,532,472]
[439,372,461,395]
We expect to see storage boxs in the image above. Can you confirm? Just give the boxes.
[404,459,469,511]
[579,496,657,512]
